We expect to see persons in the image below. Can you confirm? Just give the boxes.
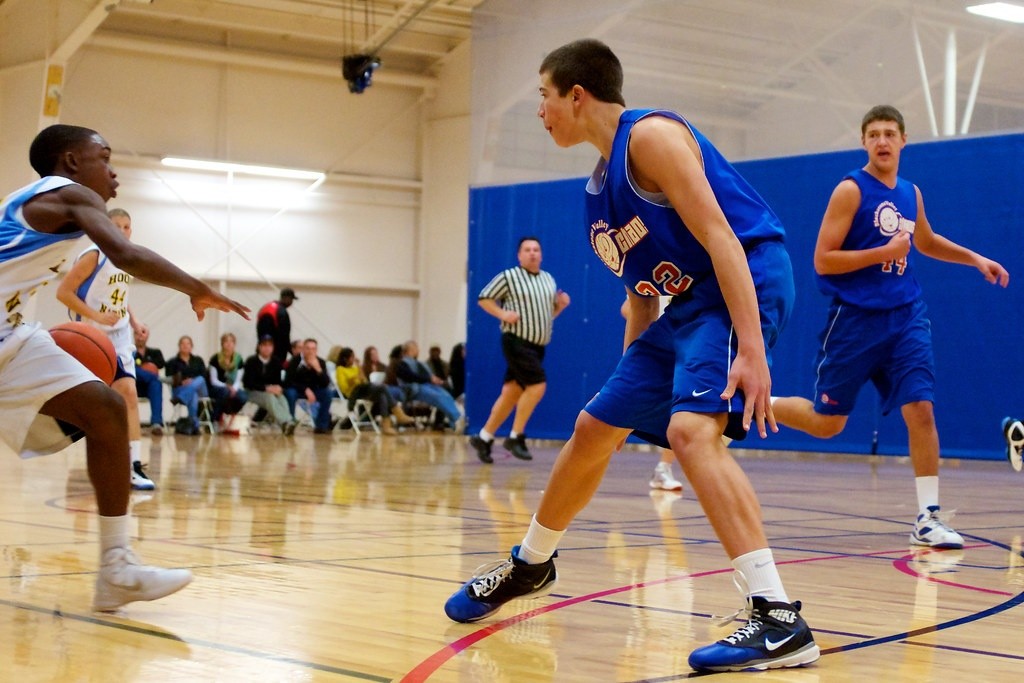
[1,123,253,611]
[445,38,820,676]
[131,324,468,437]
[55,208,154,490]
[765,104,1010,548]
[469,234,569,465]
[620,294,733,490]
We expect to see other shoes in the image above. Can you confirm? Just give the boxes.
[148,402,468,442]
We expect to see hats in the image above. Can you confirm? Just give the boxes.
[281,288,297,299]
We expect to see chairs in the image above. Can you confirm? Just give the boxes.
[137,372,437,437]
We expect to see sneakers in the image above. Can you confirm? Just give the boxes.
[911,550,963,574]
[444,545,560,624]
[909,506,965,549]
[470,433,494,464]
[446,628,558,681]
[1001,417,1024,472]
[687,569,821,673]
[649,465,682,490]
[647,491,681,517]
[502,434,533,460]
[90,547,194,613]
[130,461,155,490]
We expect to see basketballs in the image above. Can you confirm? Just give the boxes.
[48,322,118,387]
